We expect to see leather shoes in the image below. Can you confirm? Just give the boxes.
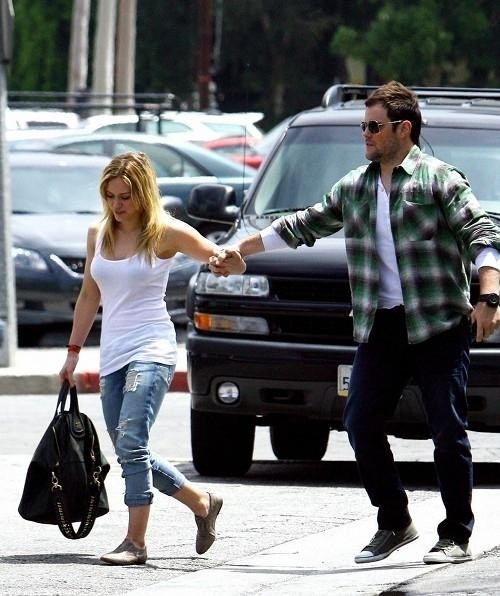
[99,537,148,566]
[193,491,225,556]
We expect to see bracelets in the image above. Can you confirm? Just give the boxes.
[66,344,82,353]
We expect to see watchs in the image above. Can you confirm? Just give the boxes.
[477,293,500,308]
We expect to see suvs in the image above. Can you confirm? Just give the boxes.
[186,83,500,477]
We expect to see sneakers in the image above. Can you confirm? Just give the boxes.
[354,519,419,564]
[423,538,473,565]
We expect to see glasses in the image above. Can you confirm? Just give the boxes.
[360,117,402,134]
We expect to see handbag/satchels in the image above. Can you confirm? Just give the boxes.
[15,374,114,542]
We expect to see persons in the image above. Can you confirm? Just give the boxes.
[209,79,500,564]
[59,150,247,565]
